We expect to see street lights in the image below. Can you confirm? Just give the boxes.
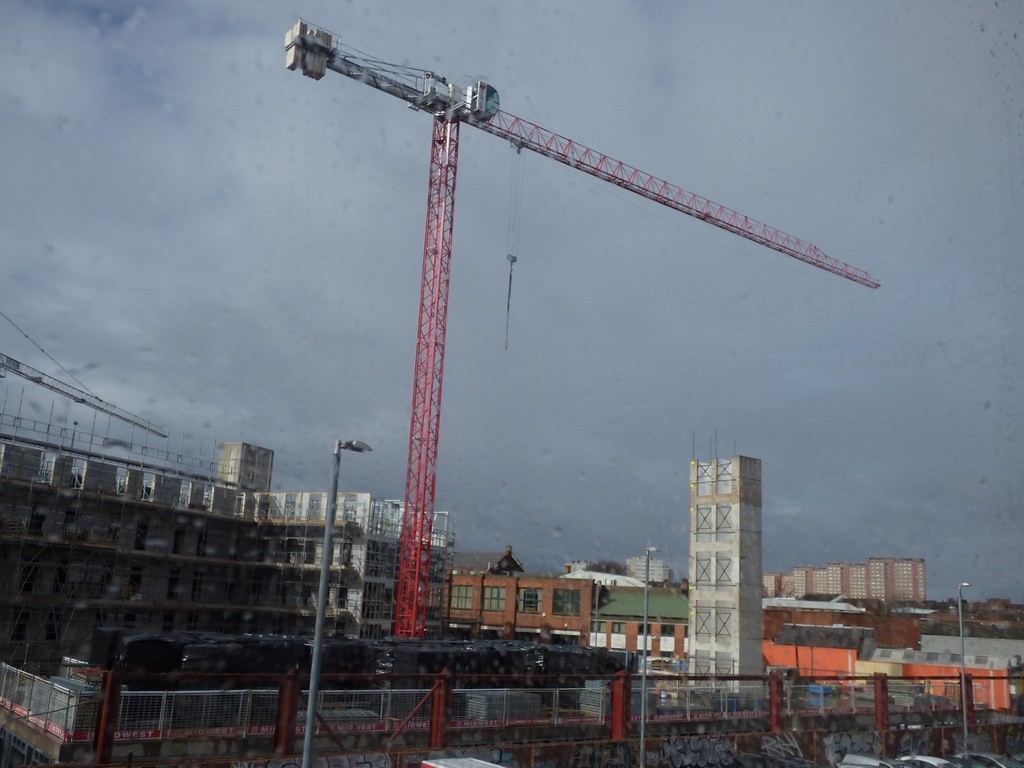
[641,545,661,765]
[302,438,373,764]
[956,582,972,759]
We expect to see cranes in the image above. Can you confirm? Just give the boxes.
[283,20,881,636]
[1,310,171,439]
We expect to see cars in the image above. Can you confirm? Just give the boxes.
[838,752,1024,768]
